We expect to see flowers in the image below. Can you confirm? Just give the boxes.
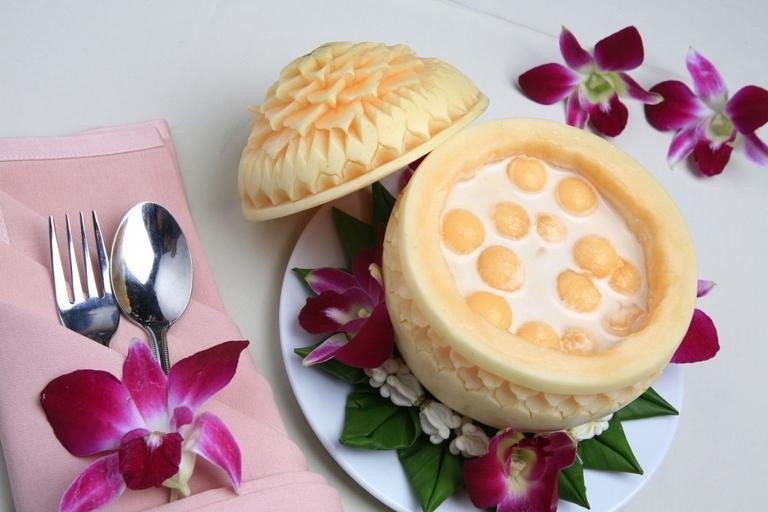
[39,339,250,510]
[516,24,664,138]
[291,235,397,370]
[642,45,768,177]
[459,424,578,511]
[668,278,721,365]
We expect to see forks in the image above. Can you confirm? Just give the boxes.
[48,209,121,346]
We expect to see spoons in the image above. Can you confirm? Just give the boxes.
[109,203,192,375]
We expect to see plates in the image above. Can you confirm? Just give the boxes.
[276,160,687,512]
